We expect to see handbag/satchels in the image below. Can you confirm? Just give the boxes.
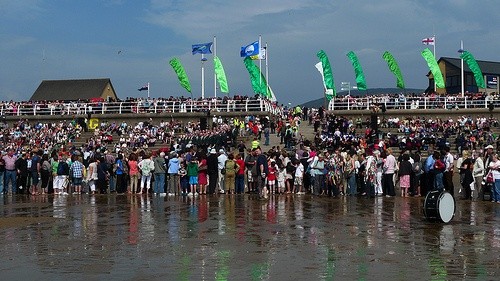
[487,172,494,183]
[464,169,474,184]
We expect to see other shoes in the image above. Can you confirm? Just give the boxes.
[386,195,390,198]
[287,191,294,193]
[296,191,306,195]
[32,192,39,196]
[59,191,70,196]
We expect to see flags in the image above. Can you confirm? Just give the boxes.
[192,41,213,54]
[250,46,266,60]
[422,36,434,45]
[240,39,259,56]
[138,82,149,90]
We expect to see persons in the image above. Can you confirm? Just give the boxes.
[0,91,500,202]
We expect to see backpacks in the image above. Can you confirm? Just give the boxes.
[141,159,152,177]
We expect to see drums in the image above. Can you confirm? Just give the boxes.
[422,187,456,224]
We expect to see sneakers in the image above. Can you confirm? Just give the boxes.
[497,200,500,203]
[187,192,193,196]
[194,192,199,195]
[72,191,82,194]
[491,199,495,203]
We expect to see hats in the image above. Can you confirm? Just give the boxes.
[484,145,494,149]
[210,149,216,153]
